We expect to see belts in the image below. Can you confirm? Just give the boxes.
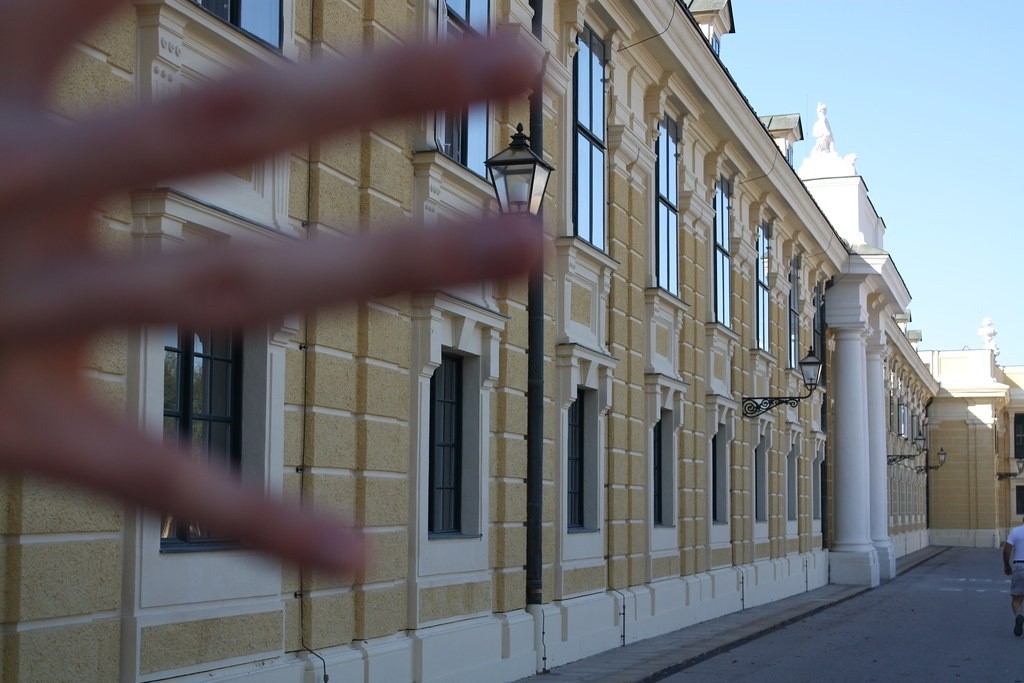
[1013,560,1024,564]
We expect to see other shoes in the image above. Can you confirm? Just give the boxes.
[1014,615,1024,636]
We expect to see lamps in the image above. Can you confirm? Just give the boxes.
[742,346,823,419]
[996,457,1024,480]
[484,123,556,216]
[887,431,927,466]
[916,447,947,474]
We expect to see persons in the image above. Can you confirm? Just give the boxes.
[1003,516,1024,637]
